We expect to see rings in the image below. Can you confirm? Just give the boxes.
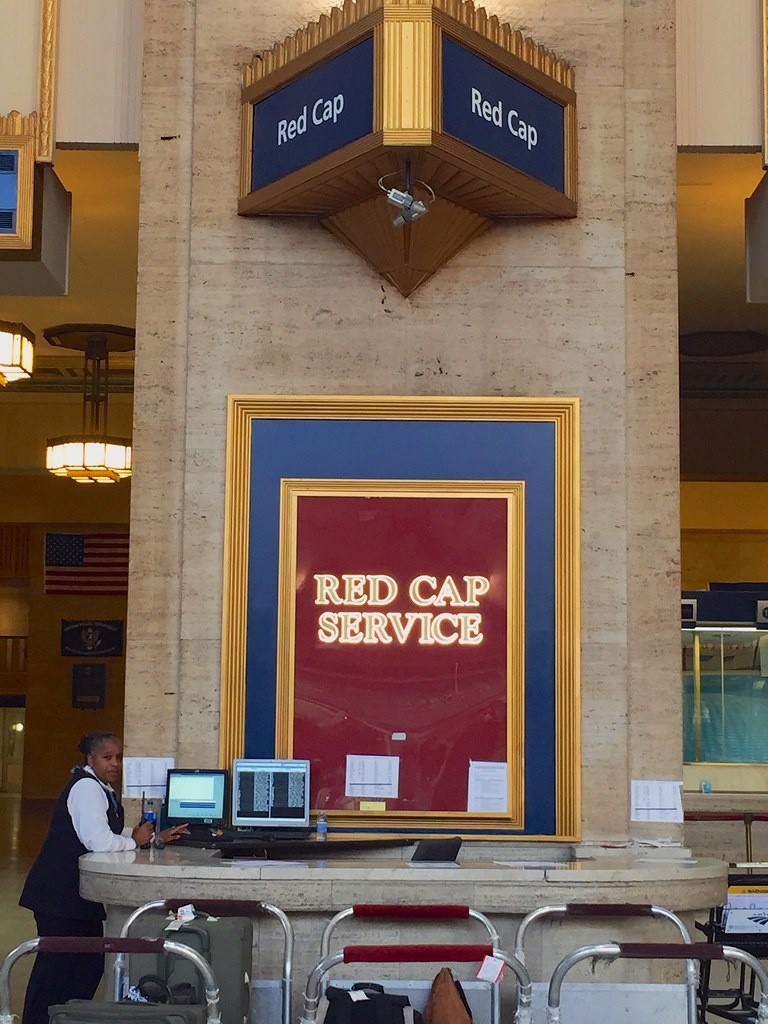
[172,826,176,828]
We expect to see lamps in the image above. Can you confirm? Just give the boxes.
[0,320,36,390]
[39,322,132,484]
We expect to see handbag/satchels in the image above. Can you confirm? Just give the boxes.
[425,966,475,1024]
[322,982,423,1024]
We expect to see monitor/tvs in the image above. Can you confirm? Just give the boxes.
[232,758,311,828]
[167,769,229,830]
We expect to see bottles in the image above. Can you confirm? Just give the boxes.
[316,811,328,842]
[143,800,157,836]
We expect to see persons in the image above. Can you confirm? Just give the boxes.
[17,732,191,1024]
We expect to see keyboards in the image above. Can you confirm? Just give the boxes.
[230,831,313,840]
